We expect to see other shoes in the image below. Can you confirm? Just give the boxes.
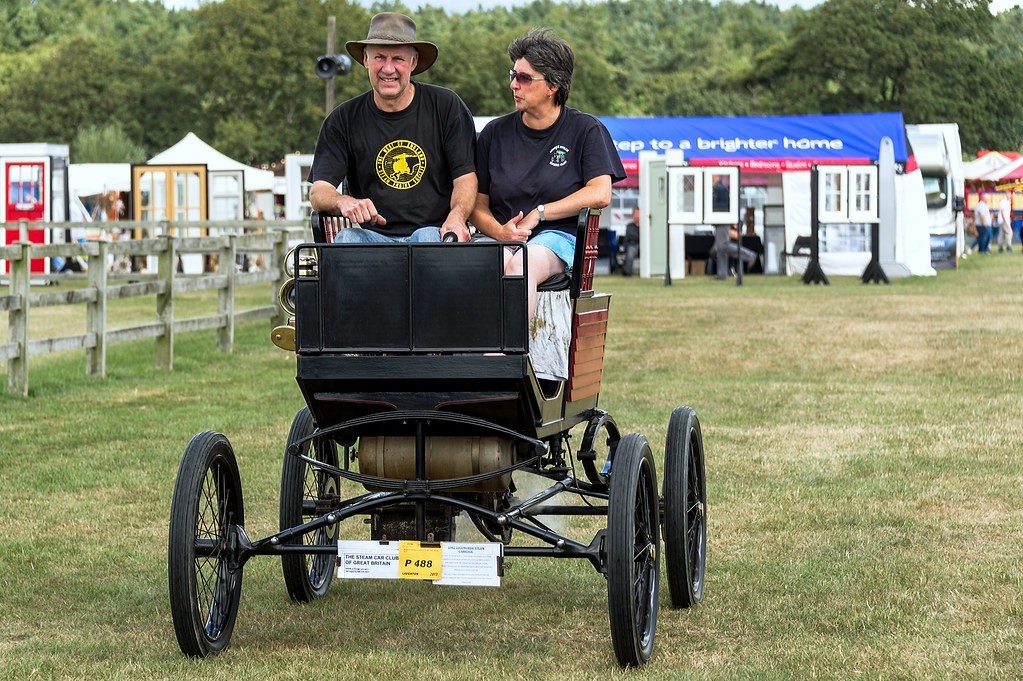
[998,247,1003,252]
[1005,246,1012,252]
[967,248,972,254]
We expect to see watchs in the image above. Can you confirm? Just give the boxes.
[537,205,545,220]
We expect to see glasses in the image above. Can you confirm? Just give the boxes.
[509,69,548,86]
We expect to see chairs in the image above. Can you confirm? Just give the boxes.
[597,227,614,273]
[684,234,716,275]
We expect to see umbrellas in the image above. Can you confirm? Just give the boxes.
[978,155,1023,182]
[964,150,1013,180]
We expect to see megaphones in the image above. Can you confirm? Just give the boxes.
[316,54,352,79]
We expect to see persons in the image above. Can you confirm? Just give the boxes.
[966,194,993,254]
[997,192,1014,252]
[622,208,640,275]
[712,175,756,279]
[470,32,627,321]
[307,12,480,242]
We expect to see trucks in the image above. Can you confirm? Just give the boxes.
[903,123,966,271]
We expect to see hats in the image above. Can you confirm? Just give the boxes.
[344,13,438,77]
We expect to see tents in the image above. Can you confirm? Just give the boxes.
[0,144,132,289]
[122,133,274,270]
[472,112,964,278]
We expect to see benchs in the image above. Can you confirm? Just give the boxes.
[310,208,603,298]
